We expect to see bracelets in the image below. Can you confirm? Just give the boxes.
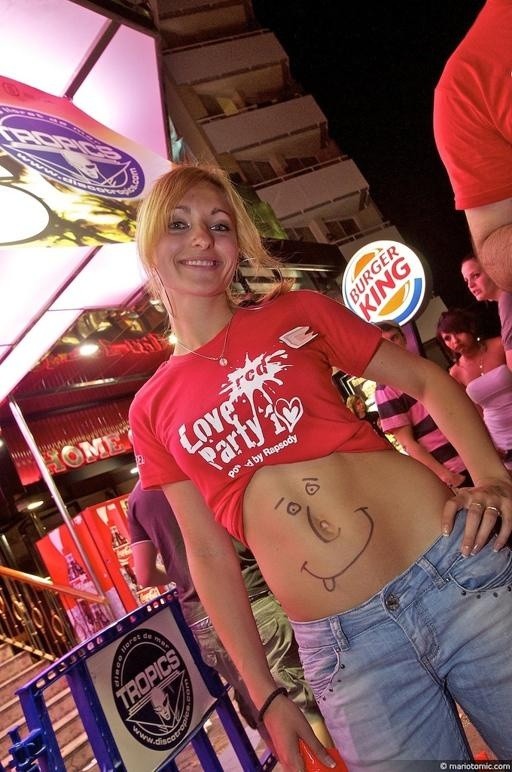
[258,687,288,722]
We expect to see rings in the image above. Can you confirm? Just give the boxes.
[486,506,501,516]
[470,501,485,509]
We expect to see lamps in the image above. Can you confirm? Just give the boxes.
[79,337,100,357]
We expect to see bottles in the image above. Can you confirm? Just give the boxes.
[62,552,108,628]
[109,522,150,593]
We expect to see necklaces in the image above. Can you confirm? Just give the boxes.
[175,315,234,366]
[479,349,485,376]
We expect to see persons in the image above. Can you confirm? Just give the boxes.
[432,0,512,294]
[346,395,393,444]
[460,256,512,374]
[374,320,476,488]
[127,426,335,772]
[129,165,512,772]
[433,311,512,472]
